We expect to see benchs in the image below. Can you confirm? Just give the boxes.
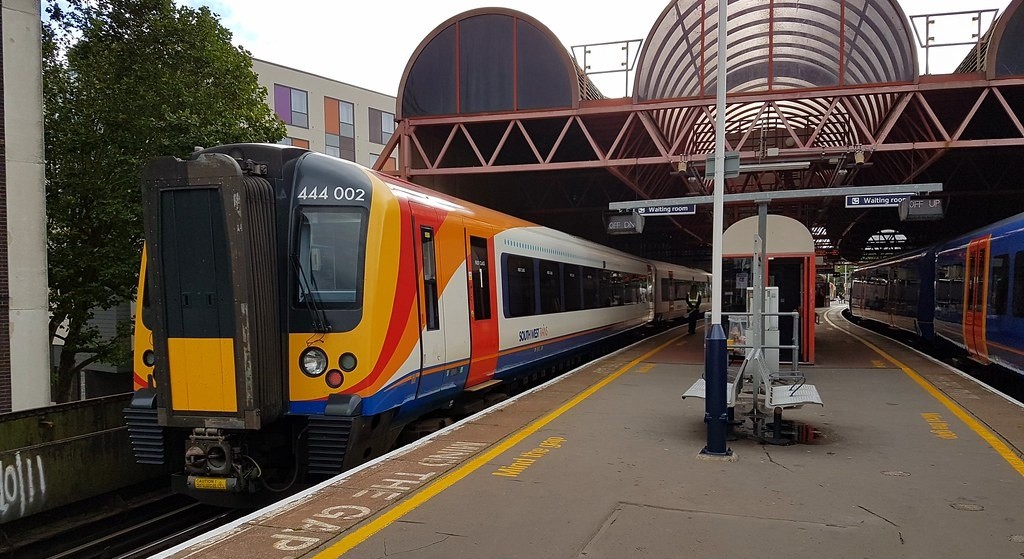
[681,348,755,440]
[753,348,825,446]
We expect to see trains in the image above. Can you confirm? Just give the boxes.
[124,142,713,506]
[848,211,1024,377]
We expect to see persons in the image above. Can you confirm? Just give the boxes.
[686,284,701,334]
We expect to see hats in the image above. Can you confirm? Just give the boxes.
[691,285,696,291]
